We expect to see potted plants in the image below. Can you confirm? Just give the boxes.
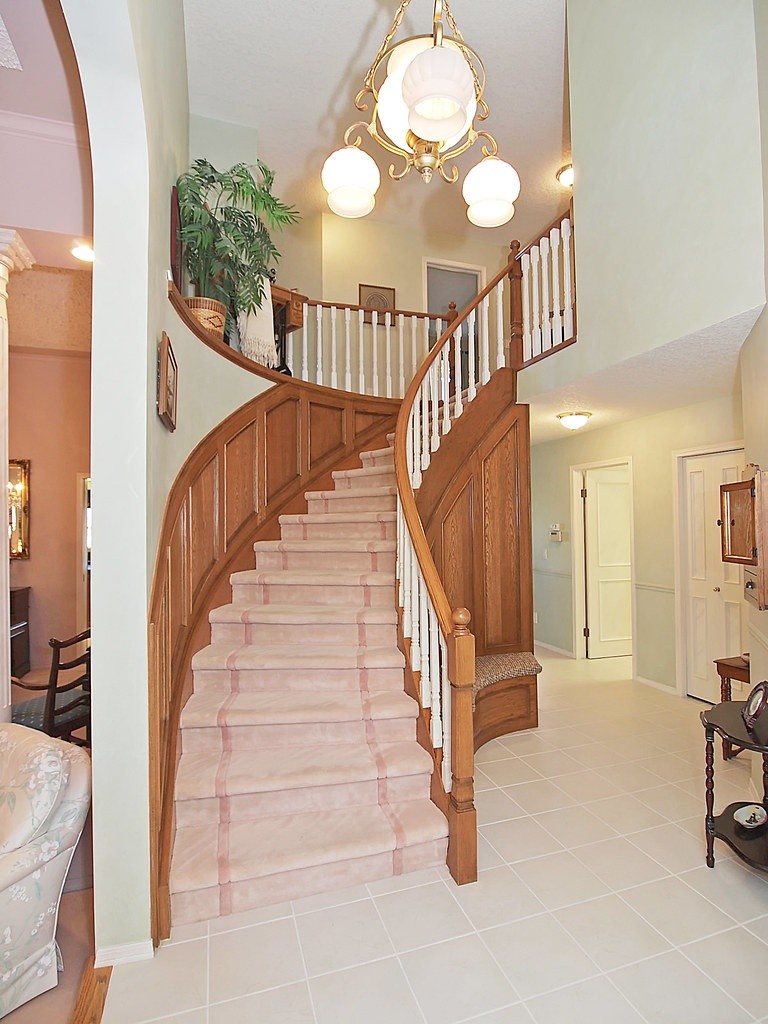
[176,157,303,342]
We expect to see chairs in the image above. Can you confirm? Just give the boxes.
[12,629,91,752]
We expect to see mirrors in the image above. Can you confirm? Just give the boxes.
[9,458,32,561]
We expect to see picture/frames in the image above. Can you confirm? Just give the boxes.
[155,330,179,433]
[358,283,396,327]
[740,681,768,729]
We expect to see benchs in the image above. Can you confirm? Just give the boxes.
[471,652,542,753]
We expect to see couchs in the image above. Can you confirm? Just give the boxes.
[0,722,91,1017]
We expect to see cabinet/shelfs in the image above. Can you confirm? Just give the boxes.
[719,463,768,612]
[700,700,768,873]
[9,585,31,678]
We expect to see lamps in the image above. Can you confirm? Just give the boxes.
[555,163,573,190]
[319,0,521,228]
[555,412,592,433]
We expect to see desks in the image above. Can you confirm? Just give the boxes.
[271,283,310,378]
[712,652,750,761]
